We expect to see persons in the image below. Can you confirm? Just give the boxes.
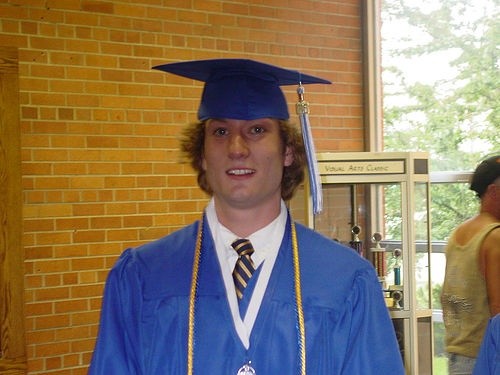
[86,57,408,375]
[439,155,500,375]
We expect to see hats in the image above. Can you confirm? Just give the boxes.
[151,59,332,215]
[470,155,500,197]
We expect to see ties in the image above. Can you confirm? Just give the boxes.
[231,238,256,304]
